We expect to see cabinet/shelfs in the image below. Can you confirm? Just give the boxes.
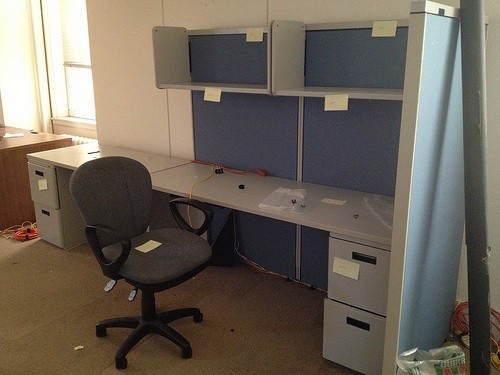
[25,0,471,375]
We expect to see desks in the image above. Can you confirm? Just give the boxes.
[0,126,73,233]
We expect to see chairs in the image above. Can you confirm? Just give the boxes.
[67,157,213,369]
